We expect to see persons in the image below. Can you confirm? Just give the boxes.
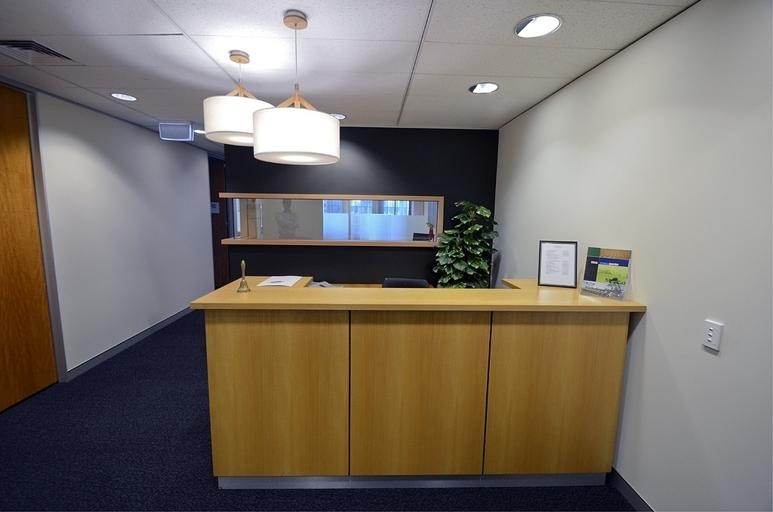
[275,200,300,236]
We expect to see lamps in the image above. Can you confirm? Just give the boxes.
[202,9,341,167]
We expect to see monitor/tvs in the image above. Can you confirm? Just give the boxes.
[413,233,434,241]
